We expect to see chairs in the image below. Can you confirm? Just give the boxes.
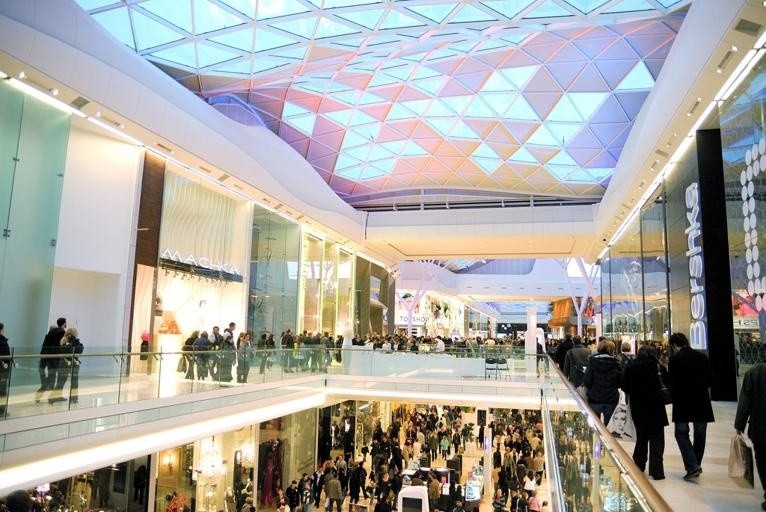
[483,354,500,380]
[497,357,510,380]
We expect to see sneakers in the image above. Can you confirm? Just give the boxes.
[35,390,80,406]
[636,465,667,481]
[257,362,329,376]
[0,410,11,419]
[683,465,702,480]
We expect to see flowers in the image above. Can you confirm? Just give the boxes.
[140,330,152,341]
[165,493,190,511]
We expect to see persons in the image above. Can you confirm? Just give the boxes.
[736,349,740,377]
[549,409,608,512]
[551,329,637,435]
[0,322,11,418]
[623,345,667,480]
[5,489,33,512]
[131,465,148,504]
[227,411,555,512]
[43,318,68,403]
[735,344,766,509]
[662,331,714,479]
[34,325,56,402]
[352,331,544,378]
[183,321,344,387]
[47,328,83,406]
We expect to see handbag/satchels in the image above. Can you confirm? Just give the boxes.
[176,355,187,374]
[728,430,755,491]
[574,382,590,402]
[656,363,673,408]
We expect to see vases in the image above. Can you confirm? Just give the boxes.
[138,341,148,359]
